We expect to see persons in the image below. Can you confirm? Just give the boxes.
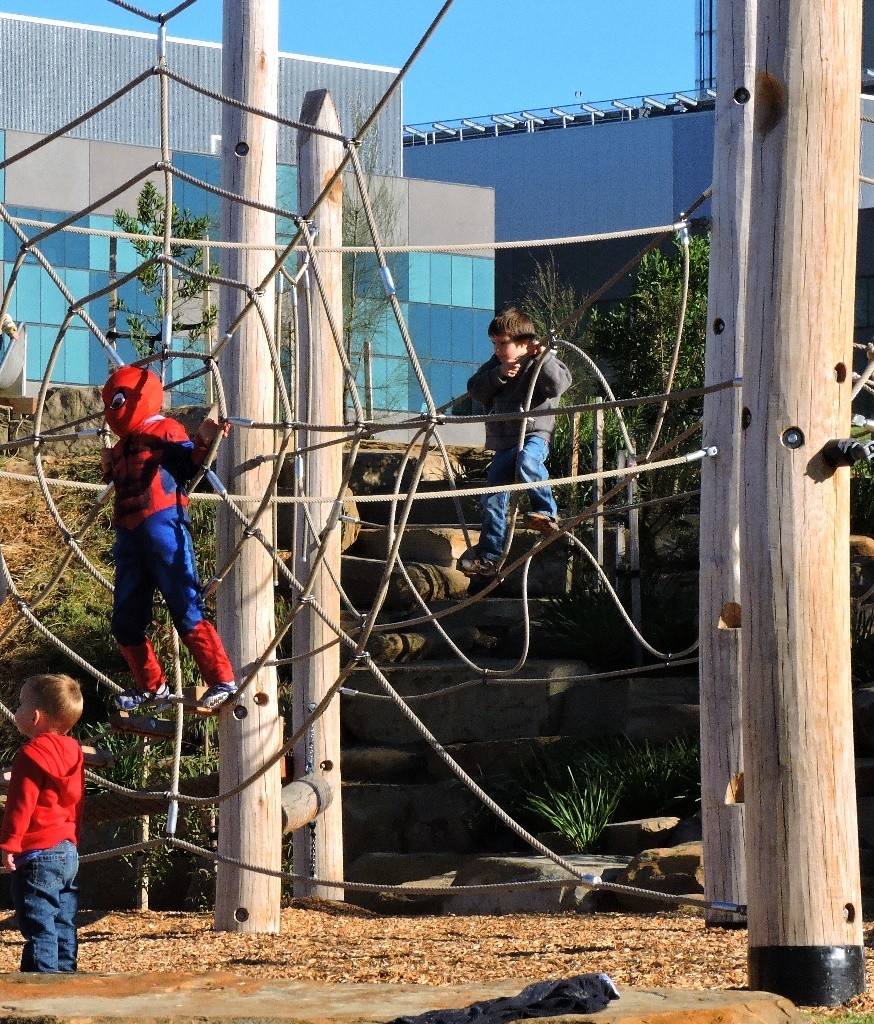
[0,674,85,973]
[461,310,572,577]
[101,368,239,710]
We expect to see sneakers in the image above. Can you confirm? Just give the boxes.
[203,683,245,709]
[523,511,560,538]
[113,683,170,710]
[460,556,497,575]
